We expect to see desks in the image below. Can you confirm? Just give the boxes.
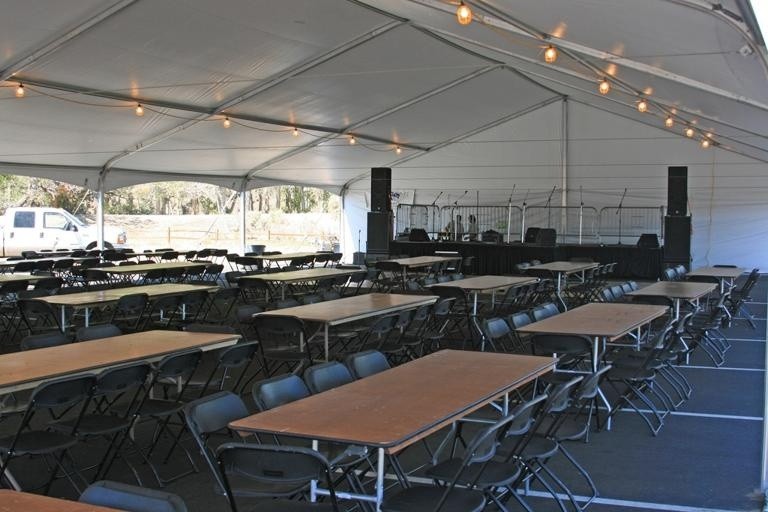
[1,329,243,490]
[515,301,670,432]
[227,348,560,511]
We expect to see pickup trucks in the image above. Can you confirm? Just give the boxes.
[1,205,128,258]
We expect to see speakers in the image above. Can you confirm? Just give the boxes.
[524,227,556,243]
[637,233,659,248]
[367,212,394,254]
[371,167,392,212]
[667,166,688,216]
[409,229,430,241]
[663,216,691,263]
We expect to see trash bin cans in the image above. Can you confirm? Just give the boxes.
[251,245,266,255]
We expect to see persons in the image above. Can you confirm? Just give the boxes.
[466,214,478,241]
[446,215,465,241]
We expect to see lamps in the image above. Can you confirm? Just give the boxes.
[14,80,26,99]
[457,0,476,26]
[222,116,401,154]
[599,75,610,96]
[135,102,143,117]
[544,43,558,63]
[638,98,710,149]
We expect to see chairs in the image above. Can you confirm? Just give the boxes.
[251,372,406,512]
[597,327,674,437]
[1,245,761,367]
[345,350,434,488]
[108,348,203,489]
[47,360,152,490]
[520,364,612,510]
[611,319,686,411]
[629,311,695,400]
[2,373,97,496]
[78,481,189,511]
[304,361,408,488]
[488,375,585,510]
[168,340,259,435]
[379,417,515,511]
[184,390,371,511]
[426,394,548,510]
